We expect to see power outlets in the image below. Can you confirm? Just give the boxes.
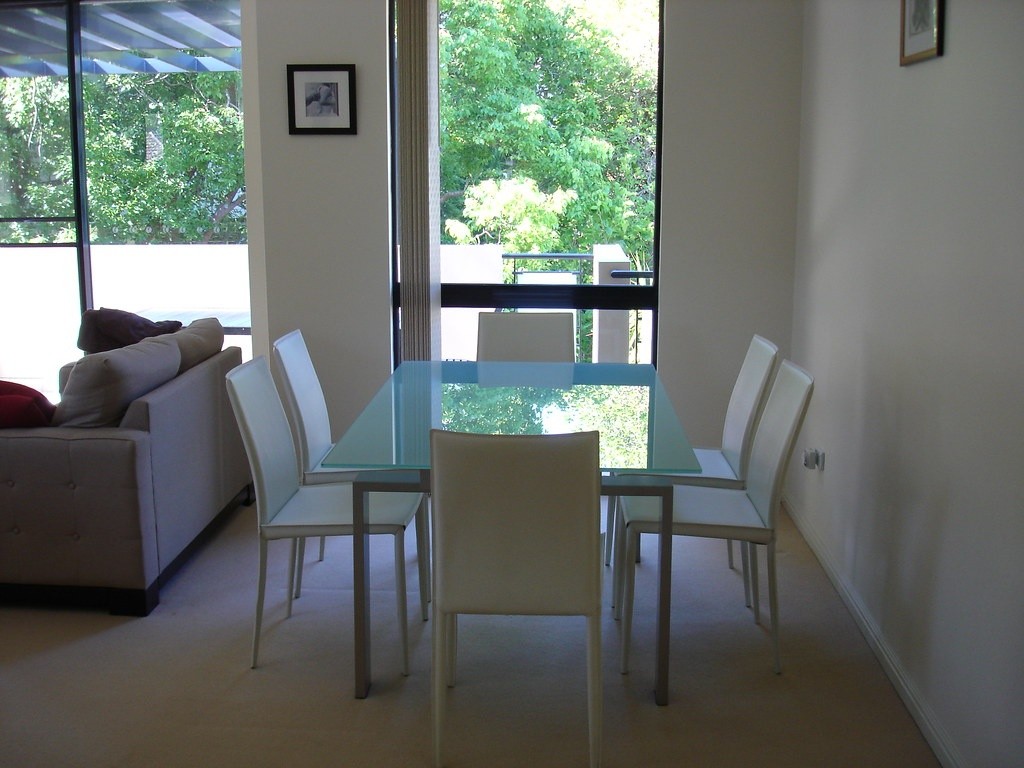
[816,450,825,471]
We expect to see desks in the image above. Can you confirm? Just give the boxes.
[320,360,703,705]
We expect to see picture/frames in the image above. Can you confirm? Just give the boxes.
[898,0,942,66]
[286,64,358,136]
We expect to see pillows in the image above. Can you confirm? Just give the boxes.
[0,394,49,428]
[0,380,56,417]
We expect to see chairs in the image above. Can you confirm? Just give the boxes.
[477,312,576,362]
[226,355,429,678]
[273,329,433,604]
[606,334,779,608]
[612,358,815,676]
[429,429,604,768]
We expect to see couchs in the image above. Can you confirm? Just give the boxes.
[0,317,255,617]
